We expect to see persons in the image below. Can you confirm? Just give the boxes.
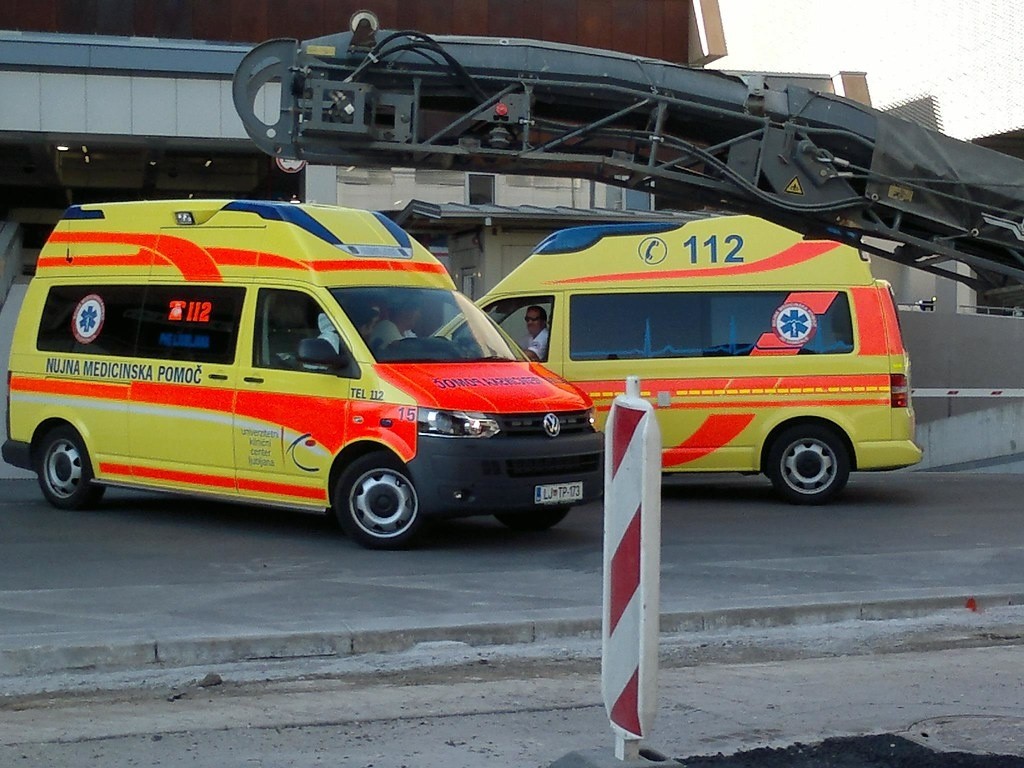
[302,288,424,370]
[522,306,549,362]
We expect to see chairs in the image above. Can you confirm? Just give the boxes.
[267,303,314,371]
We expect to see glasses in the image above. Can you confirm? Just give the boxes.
[525,316,545,323]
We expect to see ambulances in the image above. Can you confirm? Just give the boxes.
[1,198,605,550]
[430,214,926,505]
